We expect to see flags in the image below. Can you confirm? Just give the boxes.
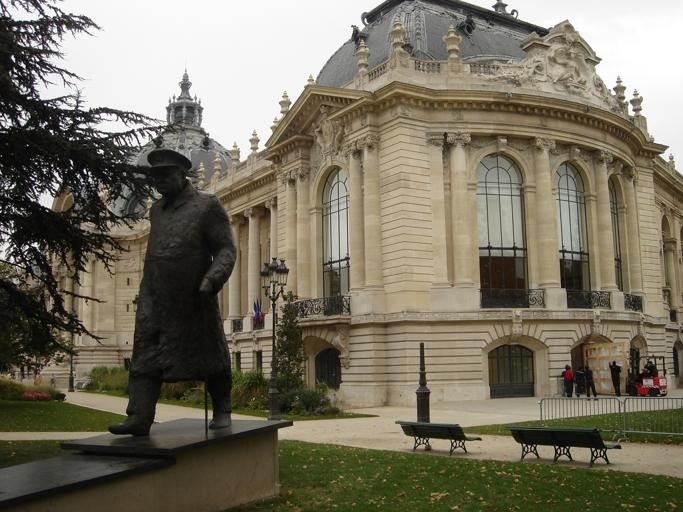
[251,299,264,321]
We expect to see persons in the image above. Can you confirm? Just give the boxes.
[108,149,236,436]
[552,48,583,83]
[50,373,56,388]
[561,360,658,400]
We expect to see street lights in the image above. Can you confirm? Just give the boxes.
[259,256,289,422]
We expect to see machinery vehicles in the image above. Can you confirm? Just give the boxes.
[627,352,668,397]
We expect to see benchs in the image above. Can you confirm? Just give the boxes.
[395,421,482,456]
[503,426,622,468]
